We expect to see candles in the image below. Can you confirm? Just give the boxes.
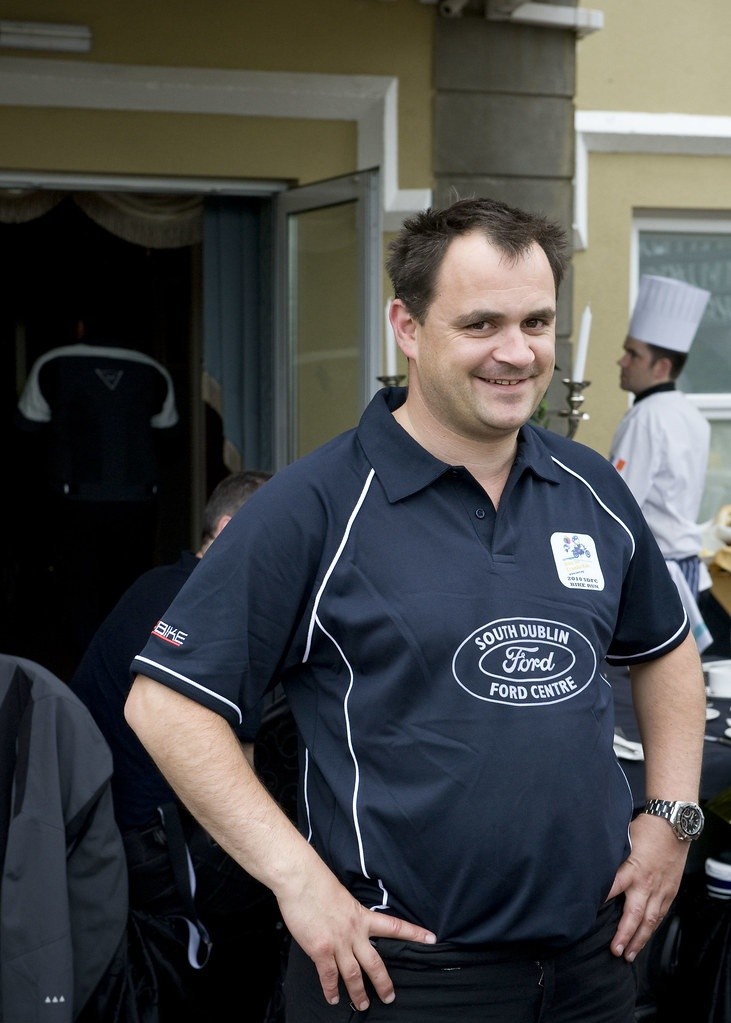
[571,306,592,382]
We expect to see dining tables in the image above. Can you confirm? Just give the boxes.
[605,651,731,811]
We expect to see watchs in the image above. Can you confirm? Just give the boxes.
[644,799,704,841]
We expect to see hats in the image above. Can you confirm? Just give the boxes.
[628,274,714,354]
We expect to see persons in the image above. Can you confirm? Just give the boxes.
[123,198,708,1023]
[77,471,303,1023]
[609,274,715,654]
